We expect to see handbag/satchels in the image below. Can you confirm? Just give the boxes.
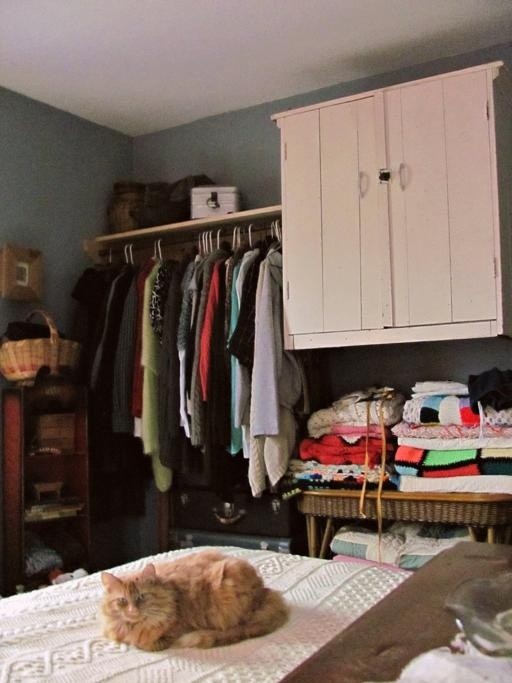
[175,485,300,537]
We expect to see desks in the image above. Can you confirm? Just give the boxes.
[297,489,512,559]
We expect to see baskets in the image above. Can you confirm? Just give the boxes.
[0,309,82,384]
[28,410,75,453]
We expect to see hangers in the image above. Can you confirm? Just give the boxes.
[86,218,281,278]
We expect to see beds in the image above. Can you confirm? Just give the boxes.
[0,543,509,683]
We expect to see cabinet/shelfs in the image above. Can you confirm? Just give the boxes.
[0,384,92,598]
[270,59,510,350]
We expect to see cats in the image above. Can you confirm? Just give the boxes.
[98,548,290,652]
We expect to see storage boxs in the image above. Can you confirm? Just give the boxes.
[191,185,240,219]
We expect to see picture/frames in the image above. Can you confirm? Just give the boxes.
[0,244,45,300]
